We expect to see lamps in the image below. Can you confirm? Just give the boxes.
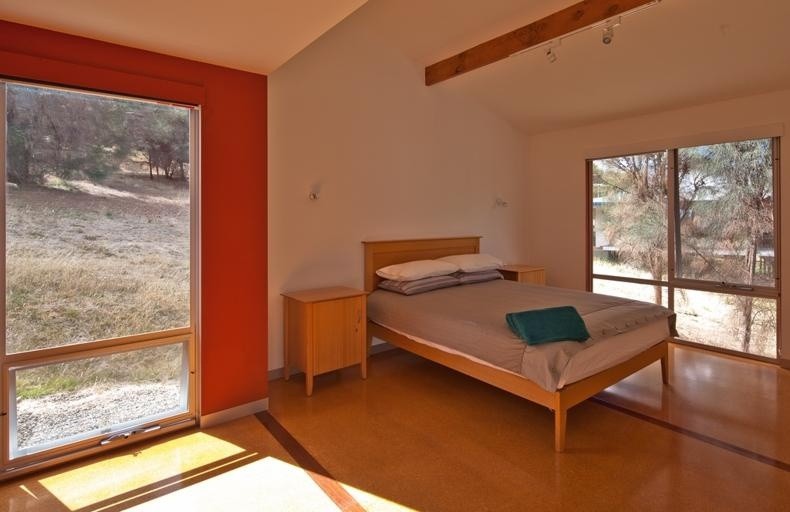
[501,6,656,63]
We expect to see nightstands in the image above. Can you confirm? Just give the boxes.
[279,284,372,397]
[495,261,549,286]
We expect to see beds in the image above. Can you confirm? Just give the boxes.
[360,234,678,453]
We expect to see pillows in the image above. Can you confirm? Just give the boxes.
[434,254,505,274]
[375,258,459,282]
[447,270,505,285]
[377,274,461,295]
